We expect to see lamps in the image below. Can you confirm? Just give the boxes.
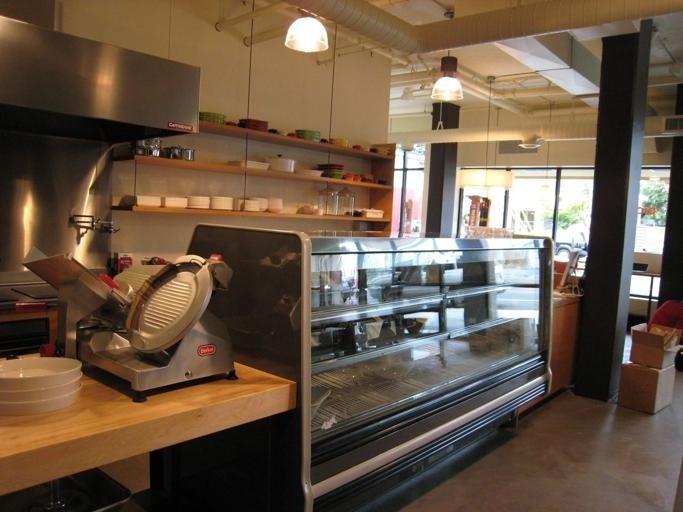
[429,3,465,100]
[284,2,330,52]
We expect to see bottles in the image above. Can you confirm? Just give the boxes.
[337,186,356,216]
[316,185,339,217]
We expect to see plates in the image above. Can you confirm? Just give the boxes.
[0,355,84,417]
[198,109,269,132]
[227,159,272,171]
[117,192,283,214]
[292,128,352,149]
[295,160,345,179]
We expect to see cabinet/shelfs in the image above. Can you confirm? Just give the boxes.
[112,120,396,237]
[186,224,554,512]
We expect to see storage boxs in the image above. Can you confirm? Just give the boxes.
[618,323,681,414]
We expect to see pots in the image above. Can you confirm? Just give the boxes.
[264,152,297,174]
